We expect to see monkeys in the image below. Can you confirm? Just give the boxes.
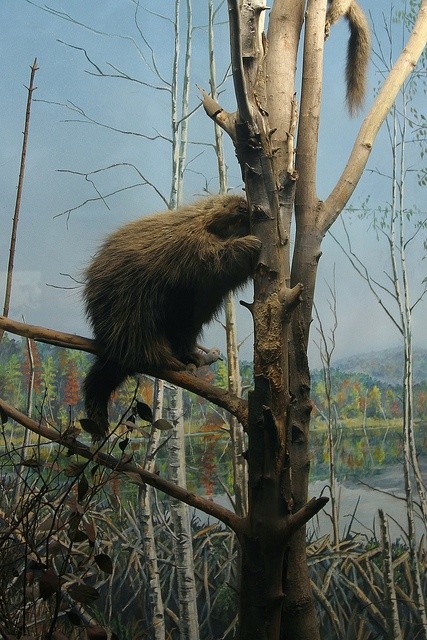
[78,192,262,445]
